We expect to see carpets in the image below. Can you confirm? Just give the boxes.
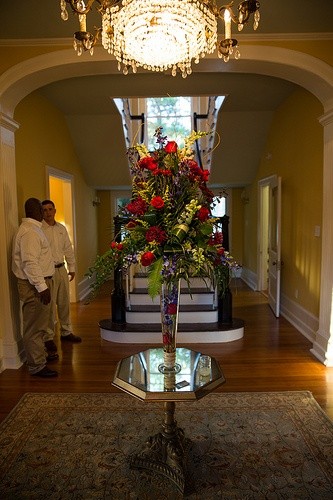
[0,390,333,500]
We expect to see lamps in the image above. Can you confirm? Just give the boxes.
[60,0,261,79]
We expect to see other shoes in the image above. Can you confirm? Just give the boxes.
[33,367,58,378]
[60,333,81,343]
[45,340,57,351]
[46,353,59,362]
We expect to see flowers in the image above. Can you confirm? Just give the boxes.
[75,121,248,306]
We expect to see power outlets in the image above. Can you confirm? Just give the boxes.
[314,224,320,237]
[293,288,298,299]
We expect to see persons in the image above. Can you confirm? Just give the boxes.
[40,200,82,351]
[11,198,58,377]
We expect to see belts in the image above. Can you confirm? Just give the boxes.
[44,276,53,280]
[55,262,65,268]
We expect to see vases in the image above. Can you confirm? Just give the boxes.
[160,248,179,367]
